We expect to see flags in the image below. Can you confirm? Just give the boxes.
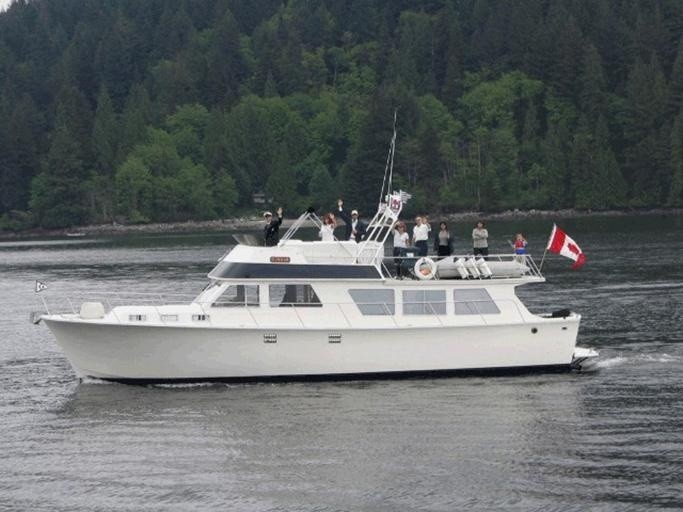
[545,222,586,272]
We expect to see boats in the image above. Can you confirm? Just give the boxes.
[42,366,584,469]
[30,108,598,388]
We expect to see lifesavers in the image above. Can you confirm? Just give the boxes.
[414,257,436,280]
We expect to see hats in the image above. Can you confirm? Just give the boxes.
[263,211,272,217]
[351,209,358,216]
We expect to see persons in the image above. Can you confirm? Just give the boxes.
[410,215,431,258]
[421,215,431,239]
[337,197,367,244]
[262,207,283,248]
[317,212,337,242]
[471,220,488,261]
[511,233,528,275]
[388,219,410,281]
[431,220,454,261]
